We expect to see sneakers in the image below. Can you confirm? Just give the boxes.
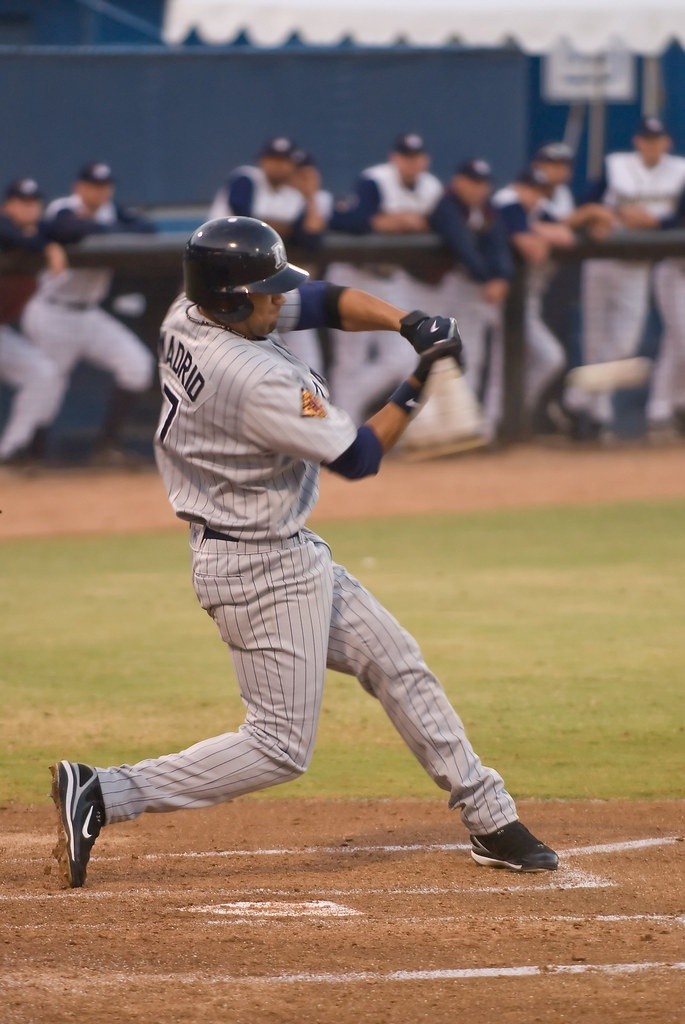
[469,821,561,872]
[46,760,106,888]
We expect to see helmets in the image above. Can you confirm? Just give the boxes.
[182,215,309,322]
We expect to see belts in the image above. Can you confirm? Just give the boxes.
[203,526,239,542]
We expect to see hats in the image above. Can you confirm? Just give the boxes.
[0,117,685,204]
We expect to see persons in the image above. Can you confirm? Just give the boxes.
[0,119,685,466]
[47,216,559,889]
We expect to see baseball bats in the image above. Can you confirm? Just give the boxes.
[409,355,483,446]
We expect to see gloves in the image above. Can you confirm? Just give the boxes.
[399,308,463,354]
[412,339,467,384]
